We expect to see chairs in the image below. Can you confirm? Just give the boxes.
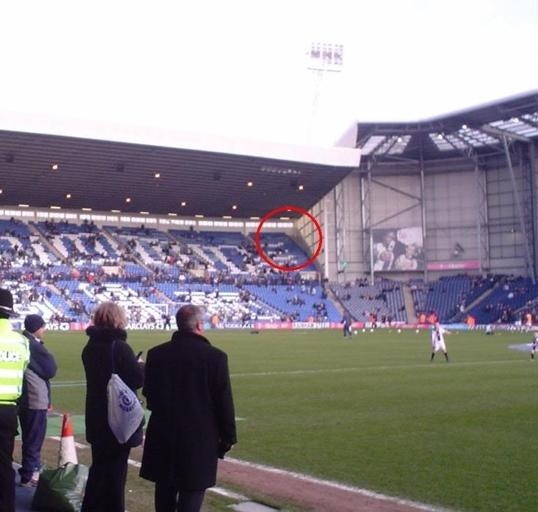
[0,217,536,325]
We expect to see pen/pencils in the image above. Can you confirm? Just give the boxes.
[136,351,143,360]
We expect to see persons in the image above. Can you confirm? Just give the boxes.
[162,312,172,330]
[392,242,420,271]
[529,329,538,360]
[1,287,30,511]
[340,271,538,337]
[137,304,238,512]
[429,320,454,361]
[373,233,396,271]
[1,215,329,324]
[17,314,60,488]
[81,301,146,512]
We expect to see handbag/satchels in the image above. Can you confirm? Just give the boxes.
[105,340,144,442]
[31,461,89,512]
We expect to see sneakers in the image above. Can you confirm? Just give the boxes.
[22,478,38,487]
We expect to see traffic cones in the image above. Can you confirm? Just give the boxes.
[58,411,78,468]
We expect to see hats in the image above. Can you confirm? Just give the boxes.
[24,314,44,332]
[1,289,16,315]
